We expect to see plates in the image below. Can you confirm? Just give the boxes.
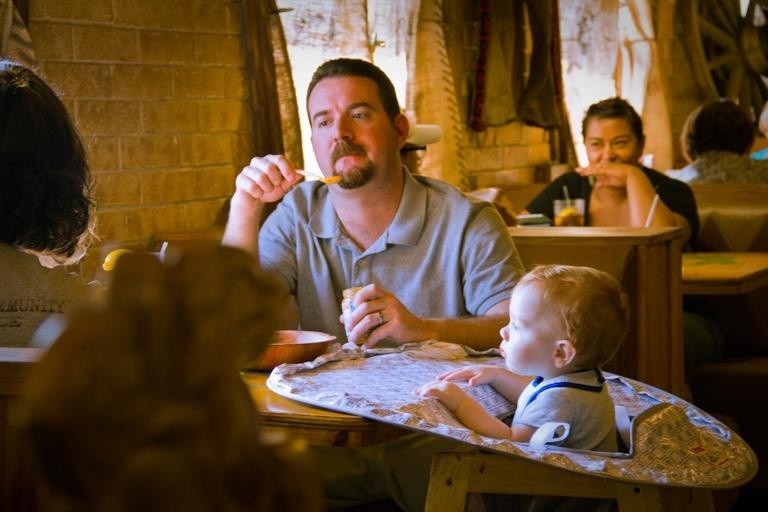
[243,330,335,372]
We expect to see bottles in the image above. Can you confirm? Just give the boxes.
[554,198,584,228]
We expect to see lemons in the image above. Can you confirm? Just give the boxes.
[102,247,132,272]
[556,206,584,224]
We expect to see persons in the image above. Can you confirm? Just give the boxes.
[398,111,442,177]
[523,98,699,252]
[0,60,98,352]
[413,264,628,452]
[223,59,525,350]
[673,98,768,185]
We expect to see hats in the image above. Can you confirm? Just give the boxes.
[404,110,442,144]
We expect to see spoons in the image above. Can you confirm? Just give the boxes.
[296,171,342,185]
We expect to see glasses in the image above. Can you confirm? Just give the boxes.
[401,146,428,158]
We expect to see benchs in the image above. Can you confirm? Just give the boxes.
[683,182,768,454]
